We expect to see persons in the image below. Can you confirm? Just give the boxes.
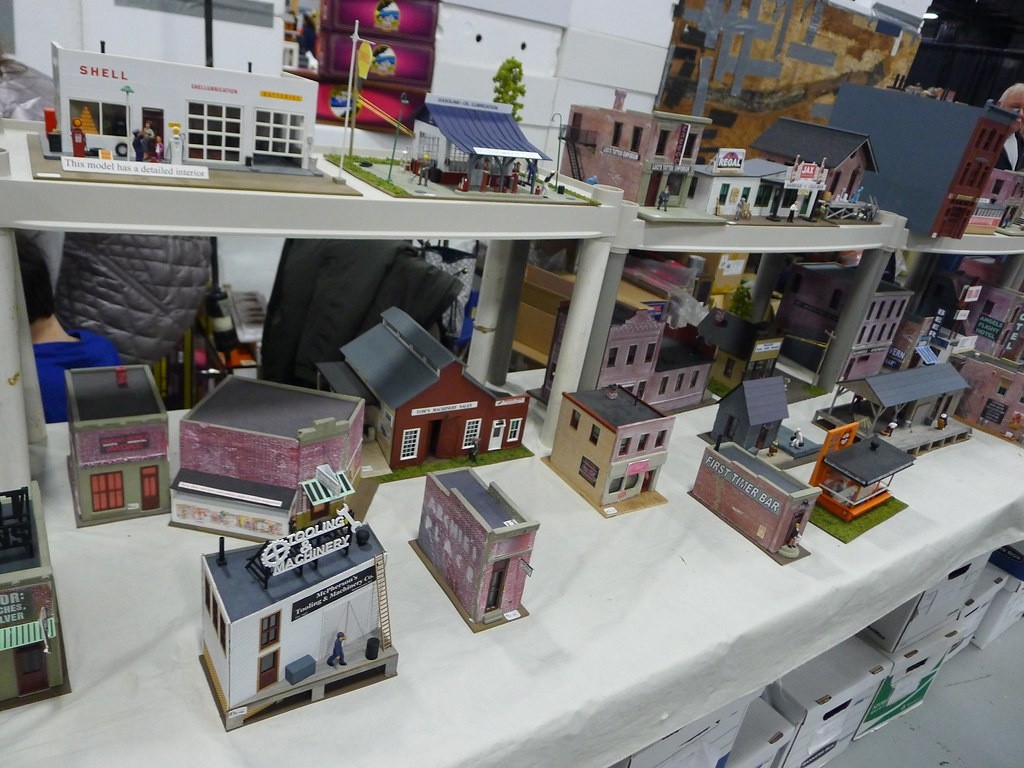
[513,163,521,172]
[417,154,430,186]
[734,197,746,220]
[327,632,347,666]
[991,83,1024,221]
[657,185,670,211]
[768,437,779,456]
[817,202,826,223]
[527,159,538,184]
[788,522,801,548]
[787,201,798,223]
[15,231,120,423]
[851,186,863,204]
[541,171,556,198]
[132,121,164,161]
[791,429,803,449]
[470,438,481,462]
[886,418,898,437]
[297,13,316,56]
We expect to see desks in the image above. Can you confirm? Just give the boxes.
[0,367,1024,768]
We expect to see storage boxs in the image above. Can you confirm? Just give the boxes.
[0,0,675,174]
[683,252,751,313]
[615,539,1024,768]
